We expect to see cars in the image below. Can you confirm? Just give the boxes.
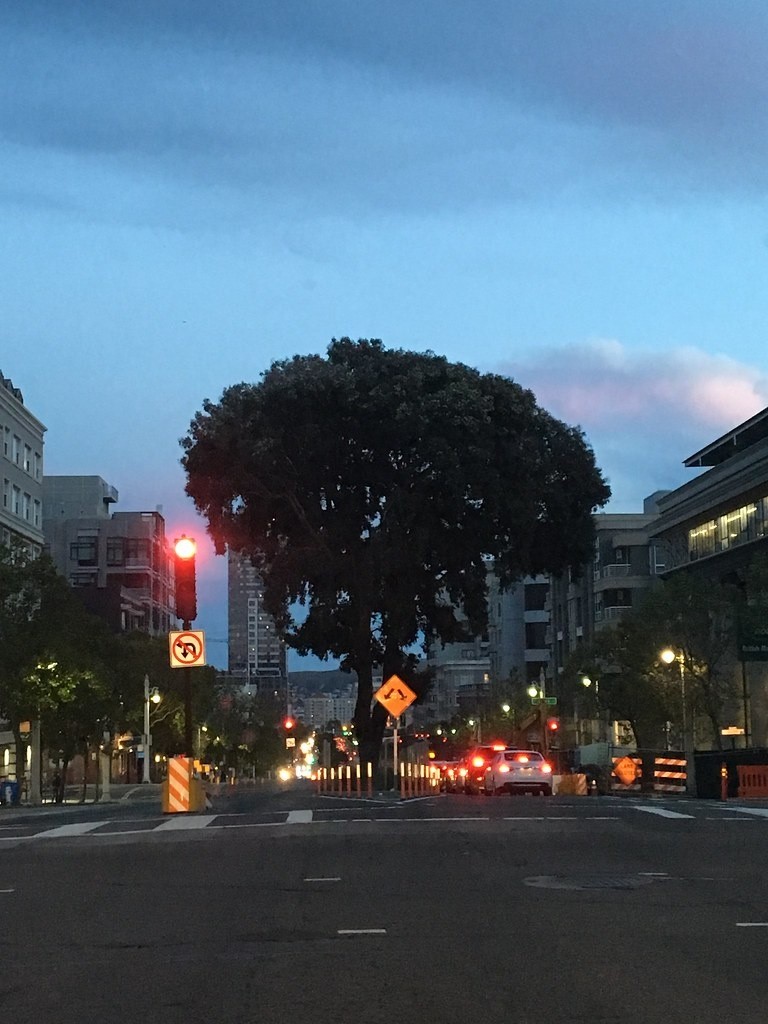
[439,745,519,796]
[483,750,552,797]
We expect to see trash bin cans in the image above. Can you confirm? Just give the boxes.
[1,782,19,808]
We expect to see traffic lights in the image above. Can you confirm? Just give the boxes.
[174,534,197,621]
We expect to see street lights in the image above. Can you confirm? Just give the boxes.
[140,674,161,784]
[660,646,699,800]
[527,666,549,759]
